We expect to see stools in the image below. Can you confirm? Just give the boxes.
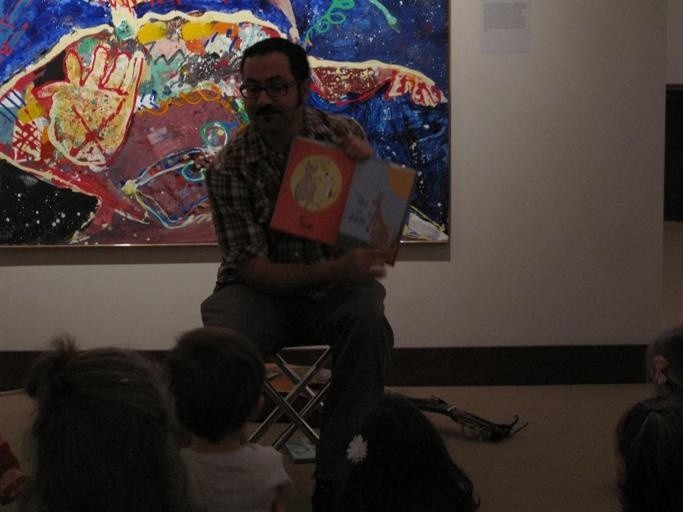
[245,327,333,453]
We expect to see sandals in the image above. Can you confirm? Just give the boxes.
[0,442,32,505]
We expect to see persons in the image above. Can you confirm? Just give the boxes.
[646,326,681,398]
[1,333,191,512]
[328,395,481,511]
[599,396,682,511]
[167,326,293,511]
[200,38,394,512]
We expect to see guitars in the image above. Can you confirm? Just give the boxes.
[250,365,528,442]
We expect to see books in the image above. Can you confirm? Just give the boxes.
[267,135,418,266]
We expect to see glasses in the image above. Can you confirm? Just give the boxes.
[239,81,297,100]
